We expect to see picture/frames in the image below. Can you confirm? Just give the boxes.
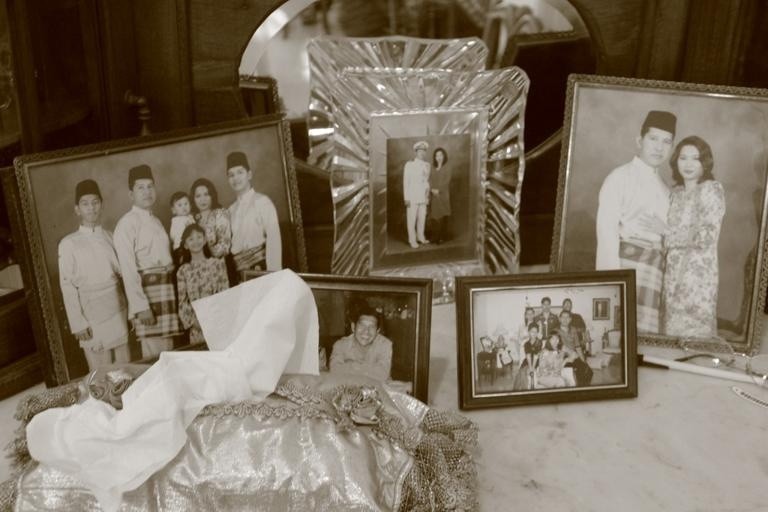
[333,61,531,311]
[301,33,484,178]
[548,73,768,356]
[10,113,312,395]
[454,268,645,412]
[238,267,434,411]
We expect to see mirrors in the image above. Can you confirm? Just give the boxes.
[185,1,650,243]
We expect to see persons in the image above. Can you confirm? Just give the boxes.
[224,151,283,286]
[639,135,727,338]
[512,322,543,390]
[176,223,230,346]
[58,180,136,372]
[113,164,179,361]
[518,307,543,343]
[403,141,431,248]
[330,311,395,388]
[428,147,452,244]
[535,328,578,388]
[168,191,195,264]
[553,311,593,387]
[560,298,590,364]
[595,110,678,336]
[534,295,559,339]
[180,179,240,288]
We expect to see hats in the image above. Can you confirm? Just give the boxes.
[413,141,429,151]
[646,109,676,133]
[75,180,103,203]
[128,165,153,189]
[227,152,250,169]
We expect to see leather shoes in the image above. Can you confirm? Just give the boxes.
[411,242,419,249]
[419,239,429,244]
